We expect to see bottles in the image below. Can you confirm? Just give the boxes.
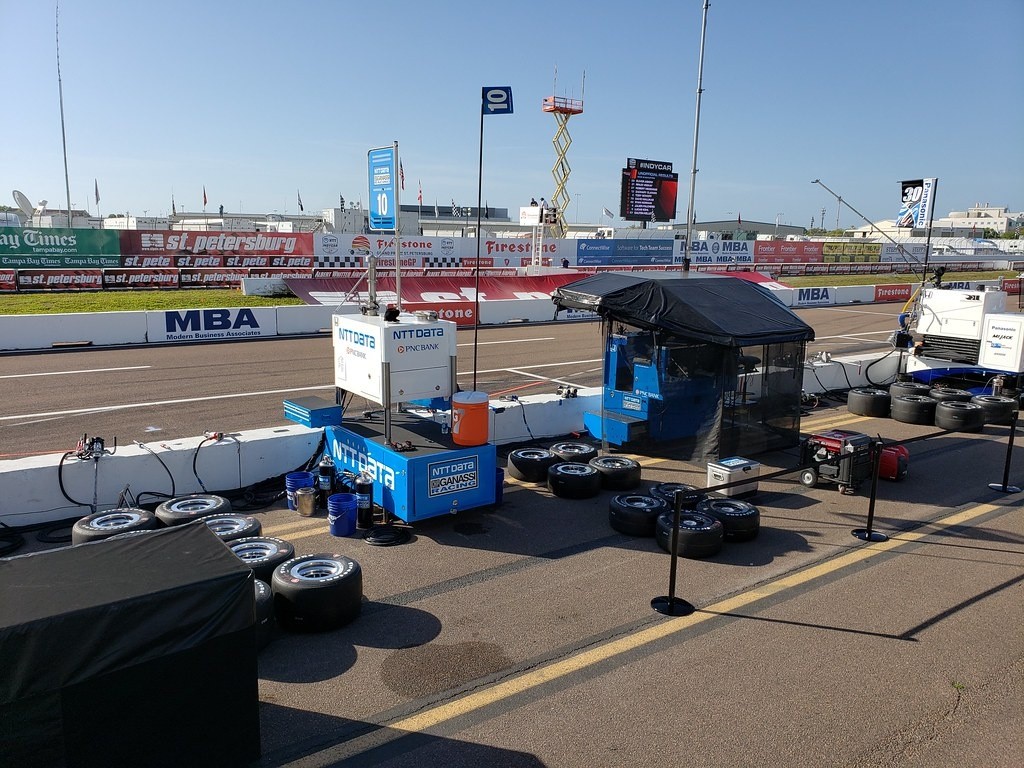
[354,471,374,528]
[319,456,336,509]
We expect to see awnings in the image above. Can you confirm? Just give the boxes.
[950,223,953,231]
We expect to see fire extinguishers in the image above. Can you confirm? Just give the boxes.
[356,470,374,529]
[319,454,335,509]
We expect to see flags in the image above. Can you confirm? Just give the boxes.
[485,200,489,219]
[738,213,742,226]
[692,209,697,223]
[972,224,976,233]
[340,194,345,213]
[400,158,404,191]
[172,201,176,217]
[298,191,303,211]
[435,198,440,219]
[204,189,207,206]
[418,180,423,207]
[452,199,461,218]
[95,180,99,204]
[811,217,814,228]
[220,205,224,216]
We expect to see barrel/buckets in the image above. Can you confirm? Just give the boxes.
[327,493,358,537]
[451,391,489,446]
[292,487,318,517]
[285,471,315,511]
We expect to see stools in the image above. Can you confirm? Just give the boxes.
[736,356,761,405]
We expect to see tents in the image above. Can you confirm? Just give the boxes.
[552,273,814,470]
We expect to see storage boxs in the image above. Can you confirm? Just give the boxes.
[707,456,760,498]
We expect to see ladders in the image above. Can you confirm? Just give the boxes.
[536,223,543,266]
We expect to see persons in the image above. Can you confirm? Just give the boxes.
[531,198,538,207]
[540,198,549,210]
